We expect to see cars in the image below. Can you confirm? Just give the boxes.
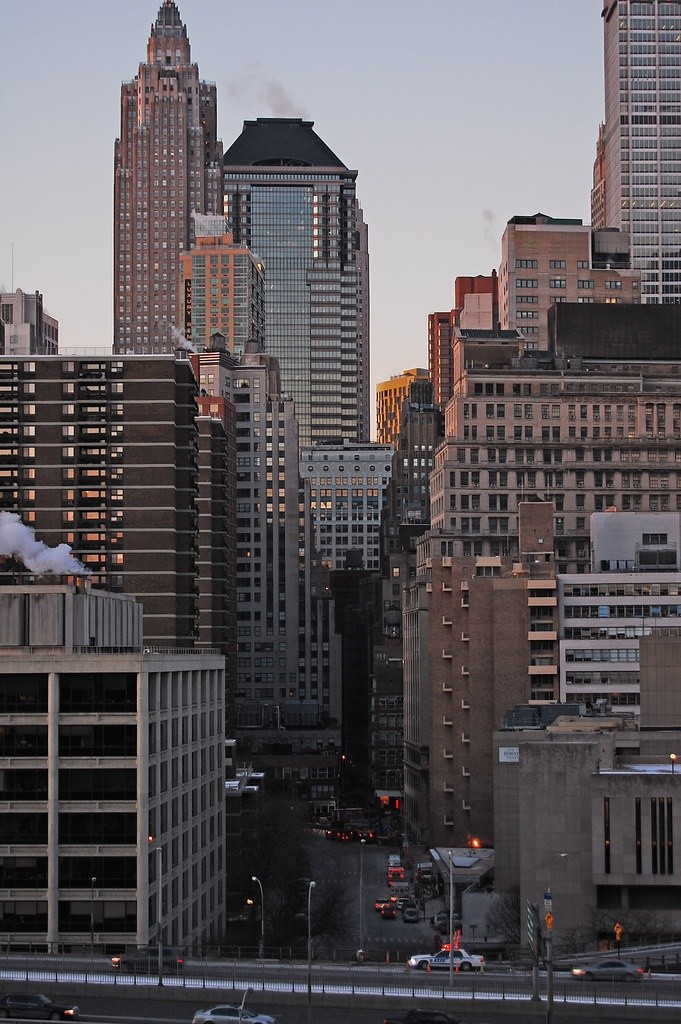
[384,1007,464,1024]
[571,958,645,983]
[191,1004,278,1024]
[408,949,486,972]
[373,853,419,924]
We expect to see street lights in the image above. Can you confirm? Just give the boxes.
[307,881,317,1024]
[91,877,97,963]
[252,876,264,958]
[447,838,480,987]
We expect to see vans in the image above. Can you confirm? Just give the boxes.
[434,913,463,930]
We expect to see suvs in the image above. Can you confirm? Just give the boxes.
[111,945,184,980]
[0,992,81,1022]
